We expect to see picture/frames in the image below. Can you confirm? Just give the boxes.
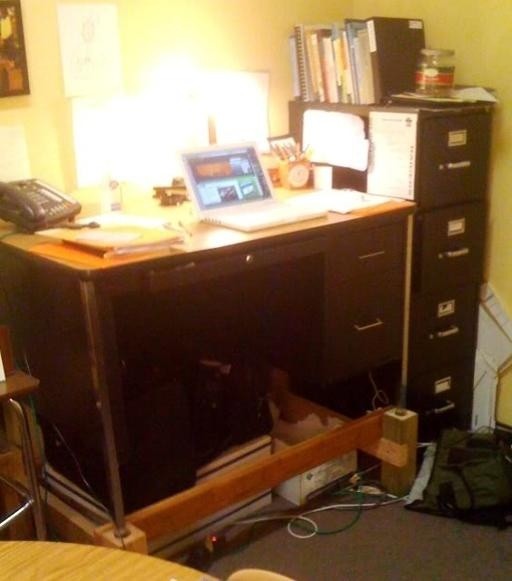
[1,1,30,100]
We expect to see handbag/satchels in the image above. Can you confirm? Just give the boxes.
[403,429,510,529]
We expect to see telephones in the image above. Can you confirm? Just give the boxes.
[1,179,82,234]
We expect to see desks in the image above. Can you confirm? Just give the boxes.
[0,189,420,560]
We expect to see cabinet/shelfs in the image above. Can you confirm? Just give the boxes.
[290,100,490,455]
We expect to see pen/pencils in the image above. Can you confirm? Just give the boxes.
[276,142,312,164]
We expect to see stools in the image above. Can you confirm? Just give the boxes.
[1,371,49,541]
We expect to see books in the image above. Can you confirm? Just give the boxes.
[33,218,184,261]
[289,15,426,106]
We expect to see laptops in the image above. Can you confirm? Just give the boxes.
[177,142,329,233]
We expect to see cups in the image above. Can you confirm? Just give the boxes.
[286,161,311,188]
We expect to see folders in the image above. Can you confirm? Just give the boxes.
[366,18,425,106]
